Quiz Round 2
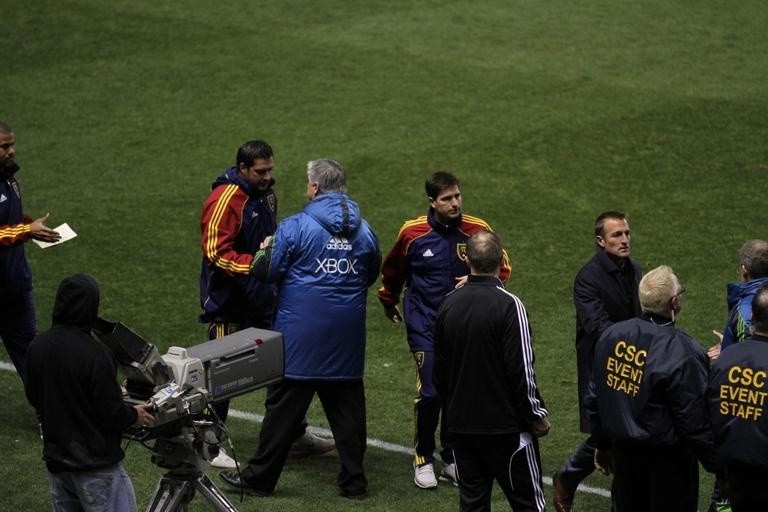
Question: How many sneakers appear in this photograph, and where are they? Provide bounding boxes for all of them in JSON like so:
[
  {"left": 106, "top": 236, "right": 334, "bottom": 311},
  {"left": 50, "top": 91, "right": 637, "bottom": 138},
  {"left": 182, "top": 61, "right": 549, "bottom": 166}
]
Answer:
[
  {"left": 291, "top": 431, "right": 337, "bottom": 456},
  {"left": 439, "top": 462, "right": 460, "bottom": 487},
  {"left": 207, "top": 447, "right": 240, "bottom": 470},
  {"left": 410, "top": 456, "right": 438, "bottom": 490}
]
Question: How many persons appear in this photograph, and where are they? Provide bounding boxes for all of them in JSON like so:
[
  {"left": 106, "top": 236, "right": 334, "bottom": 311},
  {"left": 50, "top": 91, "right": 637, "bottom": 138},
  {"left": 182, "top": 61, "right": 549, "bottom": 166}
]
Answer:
[
  {"left": 707, "top": 239, "right": 768, "bottom": 512},
  {"left": 217, "top": 158, "right": 382, "bottom": 499},
  {"left": 709, "top": 284, "right": 768, "bottom": 512},
  {"left": 198, "top": 139, "right": 336, "bottom": 468},
  {"left": 585, "top": 266, "right": 727, "bottom": 511},
  {"left": 553, "top": 211, "right": 646, "bottom": 511},
  {"left": 0, "top": 122, "right": 62, "bottom": 440},
  {"left": 378, "top": 171, "right": 513, "bottom": 490},
  {"left": 431, "top": 228, "right": 552, "bottom": 512},
  {"left": 23, "top": 272, "right": 155, "bottom": 512}
]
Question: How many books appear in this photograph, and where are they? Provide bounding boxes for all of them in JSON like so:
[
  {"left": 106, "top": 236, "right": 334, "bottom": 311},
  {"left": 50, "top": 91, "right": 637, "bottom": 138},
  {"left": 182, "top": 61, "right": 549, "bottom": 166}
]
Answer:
[{"left": 32, "top": 221, "right": 78, "bottom": 250}]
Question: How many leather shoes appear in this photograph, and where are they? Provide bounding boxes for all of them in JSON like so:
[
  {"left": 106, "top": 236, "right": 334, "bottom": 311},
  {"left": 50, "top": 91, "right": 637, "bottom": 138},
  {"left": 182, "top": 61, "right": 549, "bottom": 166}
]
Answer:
[
  {"left": 551, "top": 473, "right": 574, "bottom": 512},
  {"left": 219, "top": 470, "right": 272, "bottom": 498},
  {"left": 339, "top": 478, "right": 370, "bottom": 501}
]
